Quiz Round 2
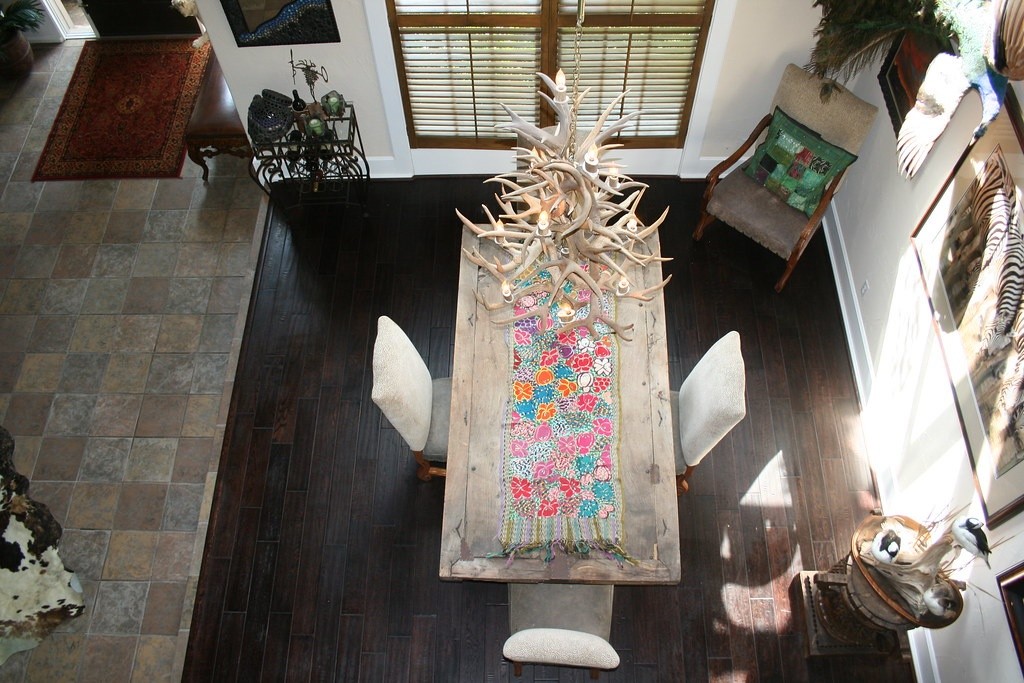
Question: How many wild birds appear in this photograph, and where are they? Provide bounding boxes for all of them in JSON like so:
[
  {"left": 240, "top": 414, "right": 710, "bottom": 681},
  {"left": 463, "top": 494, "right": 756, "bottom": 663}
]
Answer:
[
  {"left": 951, "top": 517, "right": 992, "bottom": 571},
  {"left": 872, "top": 526, "right": 902, "bottom": 564},
  {"left": 923, "top": 582, "right": 961, "bottom": 620}
]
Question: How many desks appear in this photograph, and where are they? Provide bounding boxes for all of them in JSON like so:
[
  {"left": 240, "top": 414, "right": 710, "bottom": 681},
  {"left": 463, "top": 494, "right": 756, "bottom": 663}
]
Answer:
[
  {"left": 439, "top": 222, "right": 682, "bottom": 584},
  {"left": 185, "top": 44, "right": 254, "bottom": 182}
]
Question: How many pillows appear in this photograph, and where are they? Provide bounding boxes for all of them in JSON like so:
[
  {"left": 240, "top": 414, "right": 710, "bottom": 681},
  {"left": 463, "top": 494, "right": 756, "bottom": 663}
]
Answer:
[{"left": 742, "top": 106, "right": 859, "bottom": 221}]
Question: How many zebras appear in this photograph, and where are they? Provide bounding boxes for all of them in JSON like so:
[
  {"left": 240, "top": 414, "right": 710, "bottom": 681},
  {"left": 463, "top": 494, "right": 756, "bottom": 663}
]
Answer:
[{"left": 931, "top": 150, "right": 1024, "bottom": 458}]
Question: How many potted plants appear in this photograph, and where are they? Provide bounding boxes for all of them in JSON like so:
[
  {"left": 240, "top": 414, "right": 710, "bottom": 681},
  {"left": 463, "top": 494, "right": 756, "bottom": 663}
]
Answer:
[{"left": 0, "top": 0, "right": 45, "bottom": 80}]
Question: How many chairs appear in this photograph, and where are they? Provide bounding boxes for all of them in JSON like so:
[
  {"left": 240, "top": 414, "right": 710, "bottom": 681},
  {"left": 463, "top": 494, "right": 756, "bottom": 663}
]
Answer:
[
  {"left": 670, "top": 330, "right": 748, "bottom": 496},
  {"left": 693, "top": 63, "right": 878, "bottom": 293},
  {"left": 502, "top": 583, "right": 621, "bottom": 681},
  {"left": 370, "top": 314, "right": 455, "bottom": 483}
]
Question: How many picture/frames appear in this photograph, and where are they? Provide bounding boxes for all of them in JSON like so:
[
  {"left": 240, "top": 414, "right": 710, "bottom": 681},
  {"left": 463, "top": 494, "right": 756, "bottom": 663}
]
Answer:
[
  {"left": 876, "top": 27, "right": 955, "bottom": 177},
  {"left": 908, "top": 82, "right": 1024, "bottom": 531},
  {"left": 220, "top": 0, "right": 341, "bottom": 48}
]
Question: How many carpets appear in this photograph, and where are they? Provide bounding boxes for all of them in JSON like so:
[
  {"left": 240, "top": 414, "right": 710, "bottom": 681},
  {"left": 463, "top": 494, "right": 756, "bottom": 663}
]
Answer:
[{"left": 31, "top": 35, "right": 216, "bottom": 183}]
